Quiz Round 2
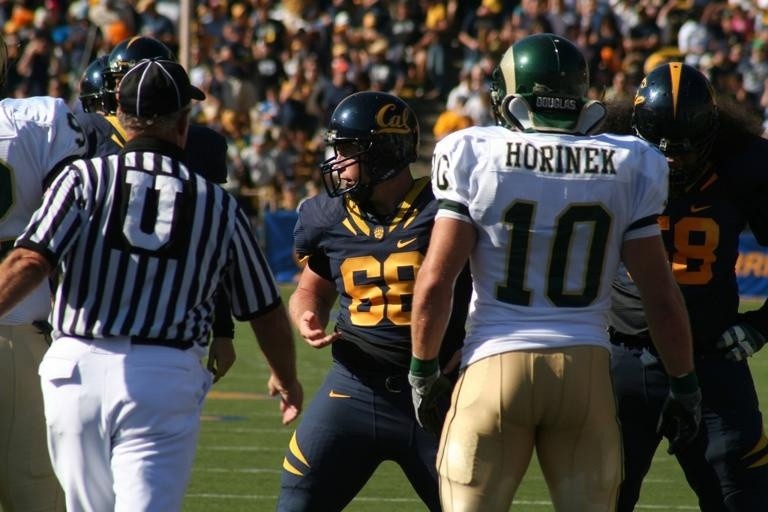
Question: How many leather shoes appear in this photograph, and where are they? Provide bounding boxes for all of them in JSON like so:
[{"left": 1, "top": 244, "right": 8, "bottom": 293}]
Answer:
[
  {"left": 657, "top": 374, "right": 702, "bottom": 454},
  {"left": 720, "top": 299, "right": 767, "bottom": 360},
  {"left": 407, "top": 358, "right": 451, "bottom": 434}
]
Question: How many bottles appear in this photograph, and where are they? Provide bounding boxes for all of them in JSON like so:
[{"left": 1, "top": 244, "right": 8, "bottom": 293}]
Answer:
[{"left": 120, "top": 57, "right": 205, "bottom": 116}]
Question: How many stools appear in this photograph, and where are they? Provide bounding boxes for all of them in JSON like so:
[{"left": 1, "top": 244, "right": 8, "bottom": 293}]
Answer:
[
  {"left": 608, "top": 326, "right": 650, "bottom": 350},
  {"left": 130, "top": 338, "right": 192, "bottom": 350},
  {"left": 378, "top": 373, "right": 406, "bottom": 397}
]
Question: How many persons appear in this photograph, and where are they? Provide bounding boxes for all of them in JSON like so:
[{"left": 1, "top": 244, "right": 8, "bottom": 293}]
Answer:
[
  {"left": 608, "top": 62, "right": 768, "bottom": 512},
  {"left": 80, "top": 56, "right": 118, "bottom": 116},
  {"left": 78, "top": 35, "right": 235, "bottom": 383},
  {"left": 2, "top": 58, "right": 304, "bottom": 512},
  {"left": 277, "top": 92, "right": 472, "bottom": 511},
  {"left": 0, "top": 96, "right": 90, "bottom": 512},
  {"left": 408, "top": 30, "right": 702, "bottom": 512},
  {"left": 0, "top": 0, "right": 767, "bottom": 208}
]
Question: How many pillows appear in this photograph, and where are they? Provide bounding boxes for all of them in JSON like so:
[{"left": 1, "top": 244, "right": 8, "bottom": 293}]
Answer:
[
  {"left": 79, "top": 56, "right": 111, "bottom": 102},
  {"left": 492, "top": 36, "right": 591, "bottom": 115},
  {"left": 107, "top": 37, "right": 177, "bottom": 74},
  {"left": 318, "top": 91, "right": 420, "bottom": 200},
  {"left": 634, "top": 62, "right": 718, "bottom": 146}
]
